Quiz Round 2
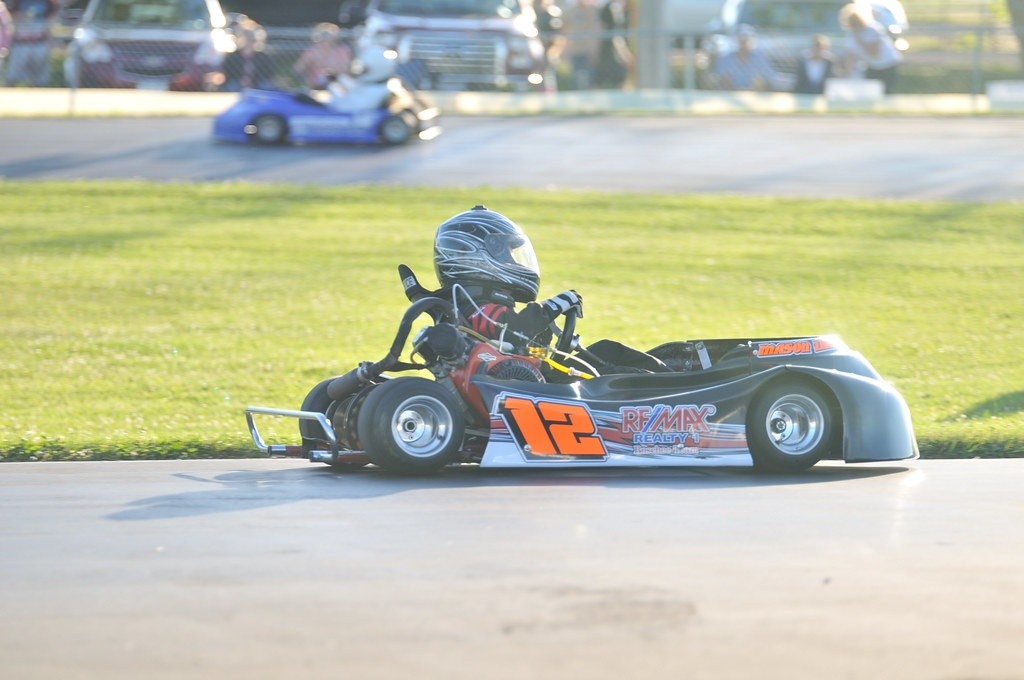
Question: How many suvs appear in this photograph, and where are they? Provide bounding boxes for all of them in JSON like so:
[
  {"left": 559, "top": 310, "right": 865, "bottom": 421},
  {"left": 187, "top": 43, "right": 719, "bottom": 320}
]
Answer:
[{"left": 338, "top": 0, "right": 563, "bottom": 94}]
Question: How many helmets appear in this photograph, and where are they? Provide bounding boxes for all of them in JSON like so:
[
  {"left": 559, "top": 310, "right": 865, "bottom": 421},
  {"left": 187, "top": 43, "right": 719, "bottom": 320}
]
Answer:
[{"left": 433, "top": 205, "right": 541, "bottom": 304}]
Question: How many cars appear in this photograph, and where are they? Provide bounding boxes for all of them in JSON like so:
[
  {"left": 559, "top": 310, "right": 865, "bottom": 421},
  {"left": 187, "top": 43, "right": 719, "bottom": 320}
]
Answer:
[
  {"left": 62, "top": 0, "right": 237, "bottom": 91},
  {"left": 706, "top": 0, "right": 902, "bottom": 96}
]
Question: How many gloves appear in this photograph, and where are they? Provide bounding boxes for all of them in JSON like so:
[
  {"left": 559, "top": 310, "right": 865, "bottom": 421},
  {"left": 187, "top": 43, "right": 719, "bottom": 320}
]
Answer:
[{"left": 541, "top": 290, "right": 583, "bottom": 320}]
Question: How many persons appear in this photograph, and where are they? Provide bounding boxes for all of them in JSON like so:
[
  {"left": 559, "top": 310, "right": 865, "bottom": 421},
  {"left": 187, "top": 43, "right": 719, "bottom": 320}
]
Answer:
[
  {"left": 0, "top": 0, "right": 910, "bottom": 114},
  {"left": 432, "top": 203, "right": 677, "bottom": 385}
]
